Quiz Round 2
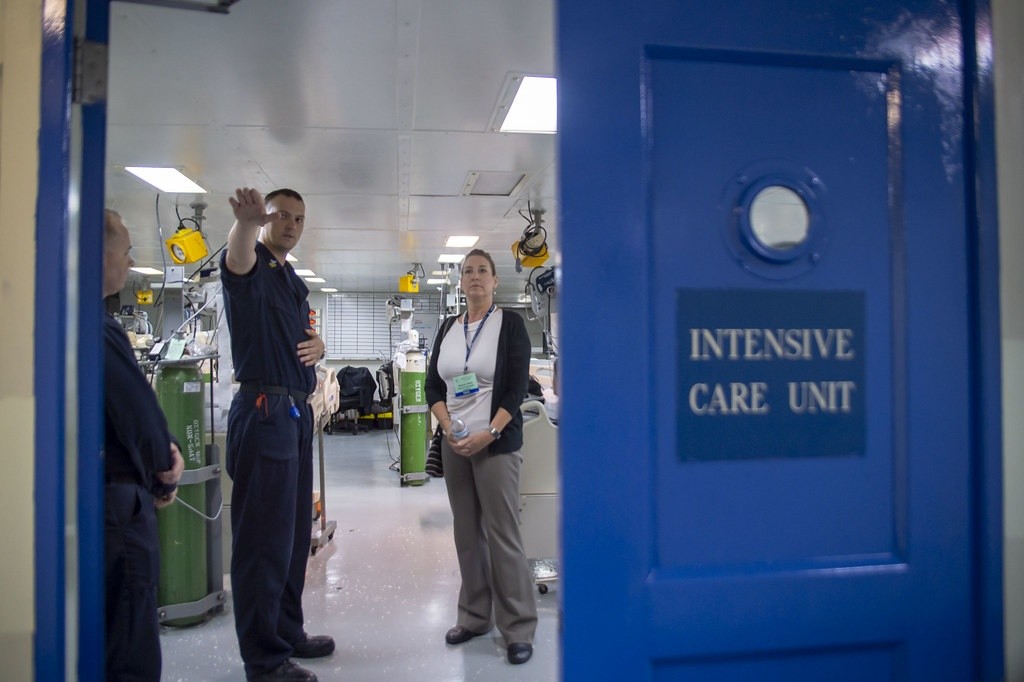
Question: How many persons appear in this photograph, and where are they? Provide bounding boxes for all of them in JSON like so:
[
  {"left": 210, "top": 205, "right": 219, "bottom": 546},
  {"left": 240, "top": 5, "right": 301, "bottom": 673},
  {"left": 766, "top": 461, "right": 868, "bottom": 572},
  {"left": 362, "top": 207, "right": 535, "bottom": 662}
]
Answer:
[
  {"left": 425, "top": 248, "right": 538, "bottom": 664},
  {"left": 219, "top": 186, "right": 335, "bottom": 682},
  {"left": 103, "top": 208, "right": 184, "bottom": 682}
]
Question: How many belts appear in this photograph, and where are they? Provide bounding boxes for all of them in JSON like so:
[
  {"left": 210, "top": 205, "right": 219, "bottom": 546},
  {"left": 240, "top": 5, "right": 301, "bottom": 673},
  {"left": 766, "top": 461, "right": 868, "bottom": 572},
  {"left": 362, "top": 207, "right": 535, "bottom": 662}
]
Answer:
[{"left": 239, "top": 383, "right": 316, "bottom": 404}]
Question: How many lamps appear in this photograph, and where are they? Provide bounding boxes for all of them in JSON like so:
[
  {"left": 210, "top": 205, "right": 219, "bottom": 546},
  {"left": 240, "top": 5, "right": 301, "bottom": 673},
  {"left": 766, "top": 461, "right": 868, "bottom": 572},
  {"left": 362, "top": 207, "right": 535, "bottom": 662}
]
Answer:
[
  {"left": 165, "top": 205, "right": 208, "bottom": 265},
  {"left": 133, "top": 280, "right": 153, "bottom": 304},
  {"left": 399, "top": 263, "right": 425, "bottom": 293},
  {"left": 511, "top": 210, "right": 549, "bottom": 267}
]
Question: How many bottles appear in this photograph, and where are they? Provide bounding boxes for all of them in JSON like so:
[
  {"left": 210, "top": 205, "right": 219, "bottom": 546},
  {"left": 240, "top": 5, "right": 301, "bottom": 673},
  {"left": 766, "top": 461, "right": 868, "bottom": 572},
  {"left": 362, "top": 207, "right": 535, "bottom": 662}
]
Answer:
[{"left": 449, "top": 414, "right": 469, "bottom": 443}]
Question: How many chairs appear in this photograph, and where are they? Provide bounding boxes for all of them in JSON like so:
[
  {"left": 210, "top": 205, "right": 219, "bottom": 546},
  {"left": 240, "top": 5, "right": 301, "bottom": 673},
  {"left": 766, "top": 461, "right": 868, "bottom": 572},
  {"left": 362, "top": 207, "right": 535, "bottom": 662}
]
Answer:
[{"left": 518, "top": 400, "right": 560, "bottom": 595}]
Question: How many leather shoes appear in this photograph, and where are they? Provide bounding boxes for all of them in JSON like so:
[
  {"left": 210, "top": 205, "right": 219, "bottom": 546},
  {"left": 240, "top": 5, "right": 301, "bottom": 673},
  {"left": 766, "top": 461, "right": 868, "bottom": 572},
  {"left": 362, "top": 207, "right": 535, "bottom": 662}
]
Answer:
[
  {"left": 248, "top": 659, "right": 318, "bottom": 682},
  {"left": 446, "top": 625, "right": 485, "bottom": 644},
  {"left": 289, "top": 632, "right": 335, "bottom": 657},
  {"left": 508, "top": 643, "right": 533, "bottom": 663}
]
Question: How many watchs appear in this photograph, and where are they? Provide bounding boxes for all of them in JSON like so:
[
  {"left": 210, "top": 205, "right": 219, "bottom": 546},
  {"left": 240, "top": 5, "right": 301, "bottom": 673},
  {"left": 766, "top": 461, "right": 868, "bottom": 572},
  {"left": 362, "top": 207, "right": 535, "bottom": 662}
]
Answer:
[
  {"left": 487, "top": 426, "right": 501, "bottom": 439},
  {"left": 320, "top": 347, "right": 326, "bottom": 359}
]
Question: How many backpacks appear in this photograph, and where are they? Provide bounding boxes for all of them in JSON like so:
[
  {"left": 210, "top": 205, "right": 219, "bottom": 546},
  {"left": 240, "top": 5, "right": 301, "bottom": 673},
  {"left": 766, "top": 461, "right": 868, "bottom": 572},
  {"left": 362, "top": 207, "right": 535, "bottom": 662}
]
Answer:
[{"left": 377, "top": 362, "right": 396, "bottom": 405}]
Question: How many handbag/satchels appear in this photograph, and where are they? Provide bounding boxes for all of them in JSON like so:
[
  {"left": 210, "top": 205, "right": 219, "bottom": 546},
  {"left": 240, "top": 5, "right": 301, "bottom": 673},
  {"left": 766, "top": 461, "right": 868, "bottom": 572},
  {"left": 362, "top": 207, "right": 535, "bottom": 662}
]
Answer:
[
  {"left": 335, "top": 366, "right": 377, "bottom": 407},
  {"left": 425, "top": 422, "right": 444, "bottom": 477}
]
